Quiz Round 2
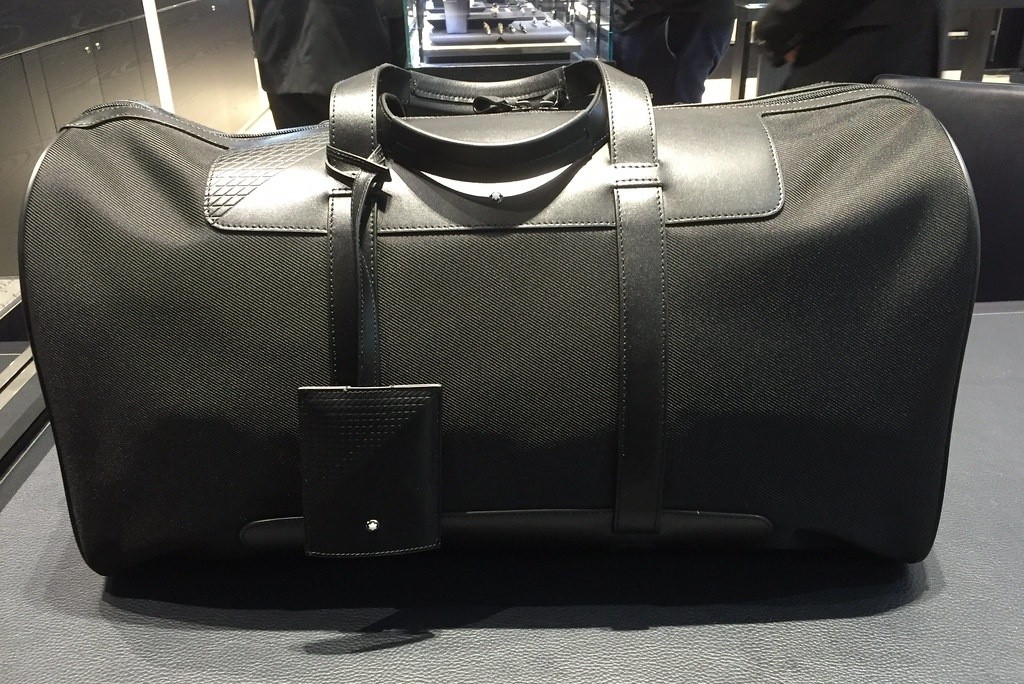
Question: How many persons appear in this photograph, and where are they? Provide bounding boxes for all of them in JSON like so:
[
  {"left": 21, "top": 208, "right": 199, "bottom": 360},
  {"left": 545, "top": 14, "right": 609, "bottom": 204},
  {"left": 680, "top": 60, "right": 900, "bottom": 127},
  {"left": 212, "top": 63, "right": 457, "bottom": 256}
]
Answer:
[
  {"left": 252, "top": 1, "right": 408, "bottom": 130},
  {"left": 610, "top": 0, "right": 736, "bottom": 106},
  {"left": 753, "top": 0, "right": 945, "bottom": 91}
]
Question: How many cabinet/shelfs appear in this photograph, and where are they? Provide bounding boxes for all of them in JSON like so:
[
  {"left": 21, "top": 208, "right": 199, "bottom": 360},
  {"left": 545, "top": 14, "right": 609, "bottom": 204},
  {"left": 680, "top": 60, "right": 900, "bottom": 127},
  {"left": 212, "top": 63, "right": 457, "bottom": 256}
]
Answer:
[{"left": 0, "top": 0, "right": 271, "bottom": 271}]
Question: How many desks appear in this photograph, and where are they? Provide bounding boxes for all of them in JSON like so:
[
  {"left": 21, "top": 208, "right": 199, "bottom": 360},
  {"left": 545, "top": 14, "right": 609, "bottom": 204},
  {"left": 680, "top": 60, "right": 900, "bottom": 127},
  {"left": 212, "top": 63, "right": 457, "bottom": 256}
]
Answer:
[{"left": 705, "top": 0, "right": 770, "bottom": 101}]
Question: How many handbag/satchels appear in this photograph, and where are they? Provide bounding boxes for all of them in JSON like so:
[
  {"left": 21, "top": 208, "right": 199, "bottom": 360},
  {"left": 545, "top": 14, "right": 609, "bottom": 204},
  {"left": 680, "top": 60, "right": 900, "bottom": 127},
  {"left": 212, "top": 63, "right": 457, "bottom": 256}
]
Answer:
[{"left": 18, "top": 58, "right": 982, "bottom": 615}]
